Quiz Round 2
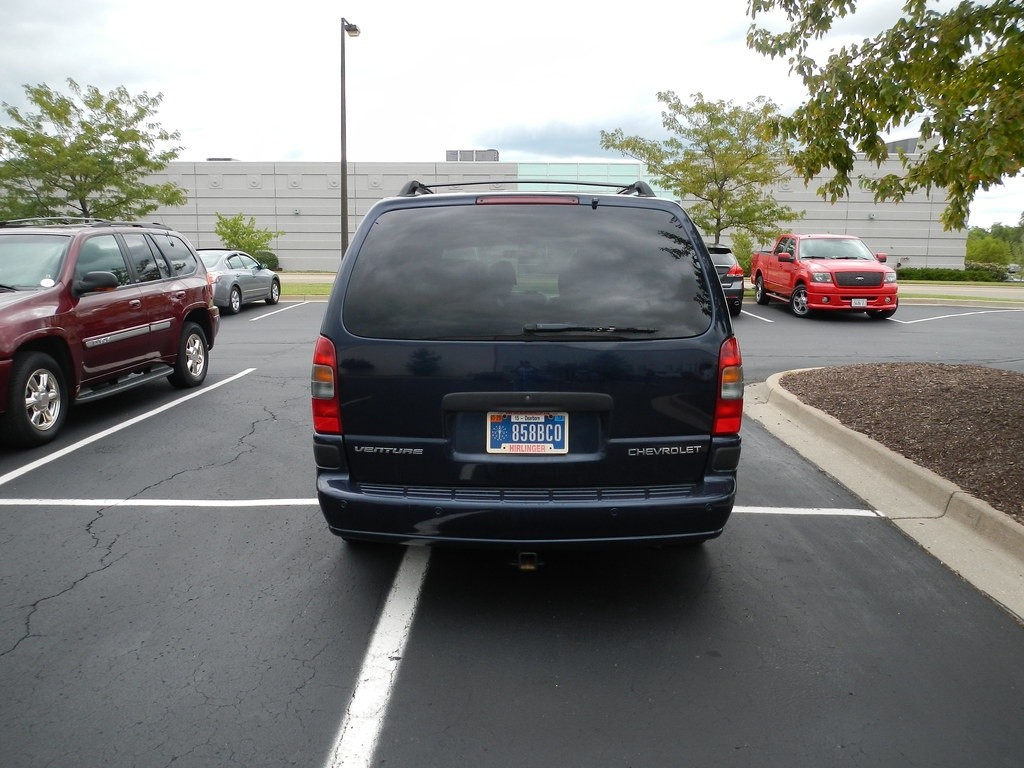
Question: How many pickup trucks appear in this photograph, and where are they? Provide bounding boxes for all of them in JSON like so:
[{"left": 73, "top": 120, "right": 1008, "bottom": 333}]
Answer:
[{"left": 750, "top": 234, "right": 900, "bottom": 320}]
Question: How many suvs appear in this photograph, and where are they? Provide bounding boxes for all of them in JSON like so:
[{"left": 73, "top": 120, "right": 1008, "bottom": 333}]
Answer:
[
  {"left": 307, "top": 177, "right": 744, "bottom": 555},
  {"left": 0, "top": 215, "right": 219, "bottom": 449}
]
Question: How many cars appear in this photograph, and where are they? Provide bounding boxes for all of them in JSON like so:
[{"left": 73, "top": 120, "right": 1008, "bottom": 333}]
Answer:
[
  {"left": 195, "top": 247, "right": 283, "bottom": 313},
  {"left": 704, "top": 245, "right": 745, "bottom": 317}
]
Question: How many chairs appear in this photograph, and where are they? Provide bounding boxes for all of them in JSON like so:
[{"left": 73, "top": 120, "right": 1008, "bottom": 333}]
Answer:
[{"left": 74, "top": 243, "right": 110, "bottom": 280}]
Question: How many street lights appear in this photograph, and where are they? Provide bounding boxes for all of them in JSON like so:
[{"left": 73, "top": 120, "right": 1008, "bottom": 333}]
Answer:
[{"left": 340, "top": 17, "right": 361, "bottom": 264}]
